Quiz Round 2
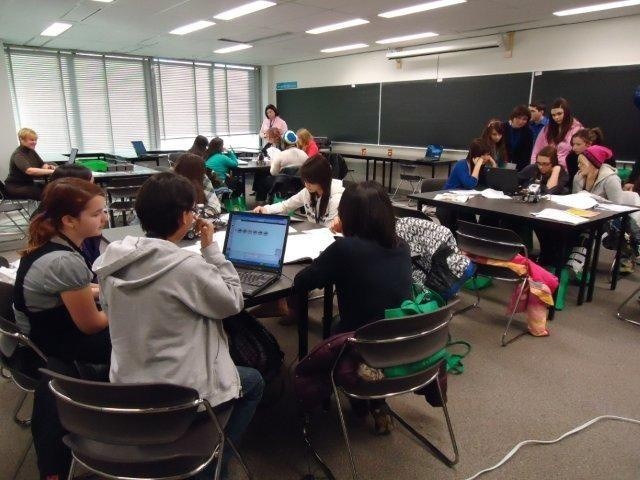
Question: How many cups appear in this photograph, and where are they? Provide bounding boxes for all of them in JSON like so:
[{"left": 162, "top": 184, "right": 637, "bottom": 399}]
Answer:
[
  {"left": 388, "top": 148, "right": 392, "bottom": 156},
  {"left": 361, "top": 148, "right": 366, "bottom": 156}
]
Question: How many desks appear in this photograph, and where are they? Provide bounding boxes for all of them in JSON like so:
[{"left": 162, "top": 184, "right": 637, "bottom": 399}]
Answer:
[
  {"left": 2, "top": 221, "right": 343, "bottom": 372},
  {"left": 407, "top": 188, "right": 640, "bottom": 320}
]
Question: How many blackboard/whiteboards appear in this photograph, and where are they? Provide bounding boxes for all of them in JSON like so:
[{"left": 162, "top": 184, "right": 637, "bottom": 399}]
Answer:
[{"left": 276, "top": 65, "right": 640, "bottom": 164}]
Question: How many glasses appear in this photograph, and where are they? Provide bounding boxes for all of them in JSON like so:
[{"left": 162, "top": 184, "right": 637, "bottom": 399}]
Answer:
[{"left": 535, "top": 162, "right": 552, "bottom": 165}]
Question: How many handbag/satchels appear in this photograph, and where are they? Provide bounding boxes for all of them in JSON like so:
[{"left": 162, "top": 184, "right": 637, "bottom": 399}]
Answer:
[
  {"left": 427, "top": 144, "right": 444, "bottom": 160},
  {"left": 222, "top": 309, "right": 285, "bottom": 405},
  {"left": 384, "top": 293, "right": 446, "bottom": 377}
]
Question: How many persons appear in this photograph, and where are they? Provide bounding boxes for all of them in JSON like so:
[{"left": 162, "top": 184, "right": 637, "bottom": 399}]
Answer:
[
  {"left": 13, "top": 164, "right": 112, "bottom": 364},
  {"left": 436, "top": 97, "right": 640, "bottom": 273},
  {"left": 6, "top": 129, "right": 59, "bottom": 200},
  {"left": 253, "top": 154, "right": 346, "bottom": 326},
  {"left": 175, "top": 135, "right": 239, "bottom": 218},
  {"left": 293, "top": 181, "right": 412, "bottom": 435},
  {"left": 249, "top": 104, "right": 318, "bottom": 206},
  {"left": 92, "top": 172, "right": 264, "bottom": 479}
]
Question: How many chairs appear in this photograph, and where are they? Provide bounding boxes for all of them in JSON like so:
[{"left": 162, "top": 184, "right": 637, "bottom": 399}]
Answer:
[
  {"left": 615, "top": 257, "right": 640, "bottom": 327},
  {"left": 2, "top": 320, "right": 48, "bottom": 430},
  {"left": 300, "top": 301, "right": 464, "bottom": 478},
  {"left": 451, "top": 220, "right": 560, "bottom": 347},
  {"left": 46, "top": 379, "right": 250, "bottom": 478}
]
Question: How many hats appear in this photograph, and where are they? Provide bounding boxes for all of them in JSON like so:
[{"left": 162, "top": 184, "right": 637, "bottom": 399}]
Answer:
[
  {"left": 283, "top": 130, "right": 297, "bottom": 145},
  {"left": 582, "top": 144, "right": 612, "bottom": 169}
]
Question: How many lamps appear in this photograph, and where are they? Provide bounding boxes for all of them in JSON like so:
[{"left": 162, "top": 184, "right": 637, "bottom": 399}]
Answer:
[{"left": 386, "top": 32, "right": 514, "bottom": 69}]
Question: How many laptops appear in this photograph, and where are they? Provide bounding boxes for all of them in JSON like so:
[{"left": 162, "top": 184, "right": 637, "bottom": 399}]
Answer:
[
  {"left": 222, "top": 210, "right": 290, "bottom": 299},
  {"left": 483, "top": 168, "right": 526, "bottom": 192},
  {"left": 131, "top": 140, "right": 160, "bottom": 157},
  {"left": 55, "top": 147, "right": 78, "bottom": 168}
]
